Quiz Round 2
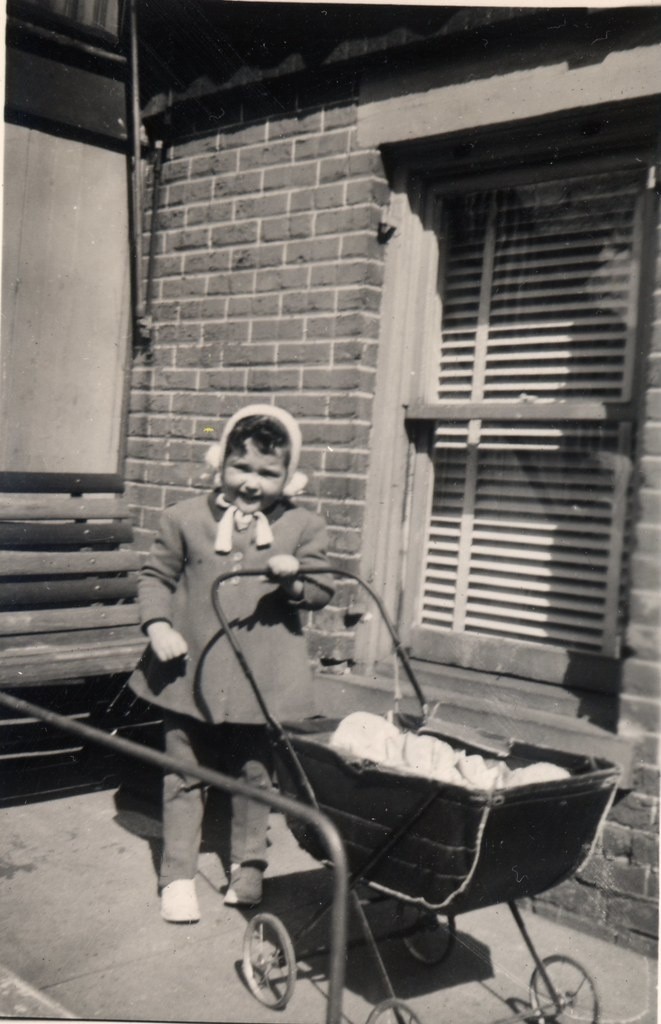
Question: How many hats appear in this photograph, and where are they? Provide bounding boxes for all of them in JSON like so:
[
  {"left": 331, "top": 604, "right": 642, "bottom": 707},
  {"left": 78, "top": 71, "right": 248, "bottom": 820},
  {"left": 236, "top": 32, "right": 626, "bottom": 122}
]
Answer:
[{"left": 200, "top": 401, "right": 310, "bottom": 555}]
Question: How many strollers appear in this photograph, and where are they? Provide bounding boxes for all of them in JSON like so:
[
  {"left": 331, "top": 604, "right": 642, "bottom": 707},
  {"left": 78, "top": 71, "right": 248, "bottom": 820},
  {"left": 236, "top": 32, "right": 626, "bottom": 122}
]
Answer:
[{"left": 209, "top": 567, "right": 623, "bottom": 1023}]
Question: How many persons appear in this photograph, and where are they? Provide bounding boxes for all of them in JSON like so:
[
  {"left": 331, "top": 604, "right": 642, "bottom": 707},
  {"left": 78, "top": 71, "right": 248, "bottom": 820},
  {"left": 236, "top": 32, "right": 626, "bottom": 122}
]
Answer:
[{"left": 128, "top": 404, "right": 335, "bottom": 922}]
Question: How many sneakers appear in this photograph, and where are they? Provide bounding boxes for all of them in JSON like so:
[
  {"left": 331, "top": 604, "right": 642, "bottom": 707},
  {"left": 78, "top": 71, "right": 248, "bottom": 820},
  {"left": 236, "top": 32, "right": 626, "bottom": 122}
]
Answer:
[
  {"left": 160, "top": 878, "right": 201, "bottom": 923},
  {"left": 221, "top": 865, "right": 266, "bottom": 907}
]
